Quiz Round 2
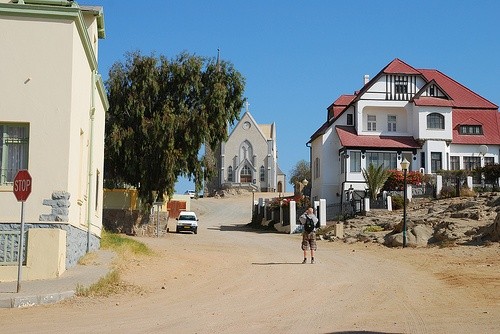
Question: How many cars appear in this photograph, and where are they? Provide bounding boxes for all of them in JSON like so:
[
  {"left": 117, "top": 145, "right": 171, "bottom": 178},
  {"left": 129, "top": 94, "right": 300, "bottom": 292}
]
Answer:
[{"left": 185, "top": 190, "right": 197, "bottom": 199}]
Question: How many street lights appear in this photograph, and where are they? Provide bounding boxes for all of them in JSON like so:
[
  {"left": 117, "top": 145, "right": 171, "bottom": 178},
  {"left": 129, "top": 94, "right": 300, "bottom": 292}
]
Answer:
[
  {"left": 302, "top": 176, "right": 309, "bottom": 212},
  {"left": 201, "top": 167, "right": 205, "bottom": 193},
  {"left": 400, "top": 156, "right": 410, "bottom": 248}
]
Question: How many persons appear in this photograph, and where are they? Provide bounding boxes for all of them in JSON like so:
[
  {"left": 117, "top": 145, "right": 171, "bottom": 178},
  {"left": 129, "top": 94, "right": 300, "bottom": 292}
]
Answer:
[{"left": 299, "top": 207, "right": 318, "bottom": 264}]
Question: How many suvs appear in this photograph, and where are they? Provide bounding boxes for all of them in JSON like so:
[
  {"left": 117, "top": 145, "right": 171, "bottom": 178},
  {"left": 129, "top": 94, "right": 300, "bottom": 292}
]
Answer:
[{"left": 176, "top": 211, "right": 199, "bottom": 233}]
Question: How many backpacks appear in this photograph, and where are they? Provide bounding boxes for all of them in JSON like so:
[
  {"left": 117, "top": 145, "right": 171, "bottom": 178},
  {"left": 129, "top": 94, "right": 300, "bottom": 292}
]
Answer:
[{"left": 304, "top": 215, "right": 314, "bottom": 234}]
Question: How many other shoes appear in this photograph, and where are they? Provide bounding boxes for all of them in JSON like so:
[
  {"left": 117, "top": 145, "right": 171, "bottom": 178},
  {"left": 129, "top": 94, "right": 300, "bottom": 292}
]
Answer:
[
  {"left": 302, "top": 260, "right": 306, "bottom": 264},
  {"left": 311, "top": 259, "right": 314, "bottom": 264}
]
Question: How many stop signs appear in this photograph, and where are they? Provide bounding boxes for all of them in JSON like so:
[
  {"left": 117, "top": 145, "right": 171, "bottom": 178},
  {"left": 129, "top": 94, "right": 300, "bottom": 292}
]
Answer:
[{"left": 13, "top": 169, "right": 33, "bottom": 202}]
